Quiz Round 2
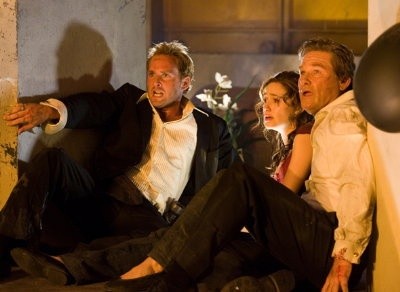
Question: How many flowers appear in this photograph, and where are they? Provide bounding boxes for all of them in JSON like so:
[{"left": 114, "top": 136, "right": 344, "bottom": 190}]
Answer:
[{"left": 197, "top": 71, "right": 241, "bottom": 114}]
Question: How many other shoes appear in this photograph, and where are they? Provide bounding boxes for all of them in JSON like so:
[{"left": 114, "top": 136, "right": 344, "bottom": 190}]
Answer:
[
  {"left": 105, "top": 269, "right": 174, "bottom": 290},
  {"left": 10, "top": 247, "right": 72, "bottom": 287}
]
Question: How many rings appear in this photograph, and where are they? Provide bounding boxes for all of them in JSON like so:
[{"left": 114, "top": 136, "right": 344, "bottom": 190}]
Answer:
[{"left": 20, "top": 106, "right": 24, "bottom": 111}]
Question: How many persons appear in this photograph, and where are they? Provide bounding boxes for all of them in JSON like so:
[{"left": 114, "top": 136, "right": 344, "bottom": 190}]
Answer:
[
  {"left": 0, "top": 39, "right": 241, "bottom": 283},
  {"left": 8, "top": 69, "right": 316, "bottom": 292},
  {"left": 137, "top": 36, "right": 378, "bottom": 292}
]
